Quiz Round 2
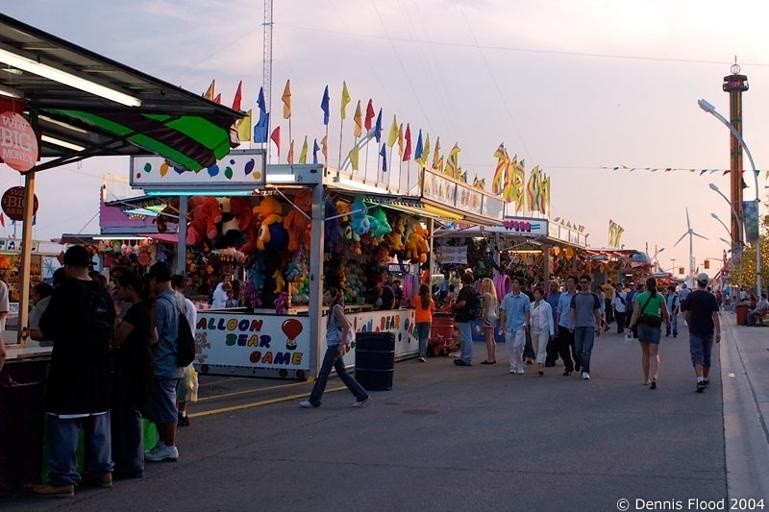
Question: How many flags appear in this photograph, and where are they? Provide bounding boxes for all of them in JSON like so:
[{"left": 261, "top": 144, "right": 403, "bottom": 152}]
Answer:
[
  {"left": 202, "top": 79, "right": 586, "bottom": 233},
  {"left": 607, "top": 220, "right": 625, "bottom": 249}
]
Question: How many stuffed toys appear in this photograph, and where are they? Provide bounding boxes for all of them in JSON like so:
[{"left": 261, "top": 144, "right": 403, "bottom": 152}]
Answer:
[{"left": 156, "top": 190, "right": 432, "bottom": 315}]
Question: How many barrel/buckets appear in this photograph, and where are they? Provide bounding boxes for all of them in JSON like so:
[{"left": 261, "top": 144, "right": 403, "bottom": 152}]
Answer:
[
  {"left": 355, "top": 332, "right": 395, "bottom": 391},
  {"left": 432, "top": 318, "right": 454, "bottom": 346}
]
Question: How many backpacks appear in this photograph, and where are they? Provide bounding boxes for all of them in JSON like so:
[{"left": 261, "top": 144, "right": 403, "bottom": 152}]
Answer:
[{"left": 470, "top": 296, "right": 480, "bottom": 319}]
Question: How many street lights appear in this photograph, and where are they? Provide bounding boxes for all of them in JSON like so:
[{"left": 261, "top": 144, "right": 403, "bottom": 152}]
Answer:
[
  {"left": 698, "top": 97, "right": 764, "bottom": 322},
  {"left": 670, "top": 258, "right": 676, "bottom": 275},
  {"left": 650, "top": 248, "right": 665, "bottom": 261},
  {"left": 339, "top": 125, "right": 384, "bottom": 172},
  {"left": 718, "top": 237, "right": 731, "bottom": 249},
  {"left": 708, "top": 183, "right": 744, "bottom": 296},
  {"left": 711, "top": 211, "right": 740, "bottom": 267}
]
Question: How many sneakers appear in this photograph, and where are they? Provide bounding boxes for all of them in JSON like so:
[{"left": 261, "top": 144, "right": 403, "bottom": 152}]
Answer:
[
  {"left": 300, "top": 400, "right": 320, "bottom": 408},
  {"left": 481, "top": 360, "right": 497, "bottom": 364},
  {"left": 583, "top": 371, "right": 590, "bottom": 380},
  {"left": 144, "top": 442, "right": 179, "bottom": 462},
  {"left": 176, "top": 411, "right": 190, "bottom": 427},
  {"left": 353, "top": 396, "right": 371, "bottom": 408},
  {"left": 33, "top": 484, "right": 75, "bottom": 498},
  {"left": 696, "top": 378, "right": 709, "bottom": 391},
  {"left": 87, "top": 470, "right": 113, "bottom": 486}
]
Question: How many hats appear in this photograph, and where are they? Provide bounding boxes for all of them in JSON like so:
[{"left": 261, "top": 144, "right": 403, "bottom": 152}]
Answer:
[
  {"left": 64, "top": 245, "right": 97, "bottom": 267},
  {"left": 696, "top": 272, "right": 708, "bottom": 282},
  {"left": 339, "top": 323, "right": 355, "bottom": 344}
]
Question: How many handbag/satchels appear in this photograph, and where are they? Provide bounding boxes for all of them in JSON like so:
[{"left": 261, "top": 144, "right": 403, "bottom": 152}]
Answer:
[
  {"left": 630, "top": 324, "right": 637, "bottom": 339},
  {"left": 178, "top": 311, "right": 196, "bottom": 367}
]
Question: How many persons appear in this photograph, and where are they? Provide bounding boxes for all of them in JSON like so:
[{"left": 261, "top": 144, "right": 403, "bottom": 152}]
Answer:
[
  {"left": 411, "top": 284, "right": 436, "bottom": 363},
  {"left": 453, "top": 268, "right": 769, "bottom": 393},
  {"left": 297, "top": 287, "right": 371, "bottom": 409},
  {"left": 0, "top": 244, "right": 199, "bottom": 498}
]
